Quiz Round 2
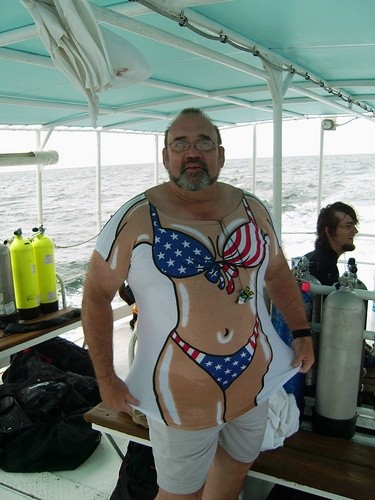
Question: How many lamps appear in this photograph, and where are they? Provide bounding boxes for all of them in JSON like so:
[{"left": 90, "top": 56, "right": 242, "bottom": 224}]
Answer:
[{"left": 321, "top": 116, "right": 360, "bottom": 131}]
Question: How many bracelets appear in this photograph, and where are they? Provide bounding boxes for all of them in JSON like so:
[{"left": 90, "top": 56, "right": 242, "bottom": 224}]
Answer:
[{"left": 292, "top": 328, "right": 313, "bottom": 339}]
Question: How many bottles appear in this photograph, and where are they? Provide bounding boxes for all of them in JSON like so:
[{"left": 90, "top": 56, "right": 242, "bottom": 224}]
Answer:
[{"left": 300, "top": 282, "right": 312, "bottom": 322}]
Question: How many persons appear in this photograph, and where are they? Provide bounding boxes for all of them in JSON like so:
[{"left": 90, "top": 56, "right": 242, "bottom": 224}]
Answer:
[
  {"left": 293, "top": 201, "right": 358, "bottom": 285},
  {"left": 79, "top": 107, "right": 316, "bottom": 500}
]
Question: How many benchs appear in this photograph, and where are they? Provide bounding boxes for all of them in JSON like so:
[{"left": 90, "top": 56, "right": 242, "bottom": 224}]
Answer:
[
  {"left": 0, "top": 273, "right": 83, "bottom": 368},
  {"left": 83, "top": 401, "right": 375, "bottom": 500}
]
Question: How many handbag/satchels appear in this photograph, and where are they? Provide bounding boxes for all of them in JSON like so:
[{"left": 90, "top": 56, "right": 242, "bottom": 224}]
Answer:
[{"left": 0, "top": 336, "right": 102, "bottom": 473}]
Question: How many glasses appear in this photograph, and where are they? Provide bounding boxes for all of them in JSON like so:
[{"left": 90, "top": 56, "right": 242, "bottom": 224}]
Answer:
[{"left": 168, "top": 140, "right": 214, "bottom": 153}]
